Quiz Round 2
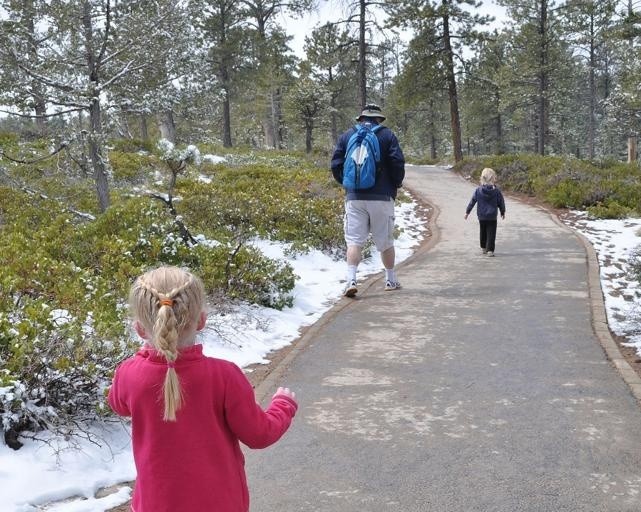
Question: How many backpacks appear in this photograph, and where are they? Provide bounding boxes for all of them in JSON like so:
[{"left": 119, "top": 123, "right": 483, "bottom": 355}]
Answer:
[{"left": 342, "top": 125, "right": 388, "bottom": 191}]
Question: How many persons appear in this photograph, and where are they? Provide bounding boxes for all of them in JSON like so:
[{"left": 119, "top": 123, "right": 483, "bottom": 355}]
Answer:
[
  {"left": 464, "top": 167, "right": 506, "bottom": 257},
  {"left": 331, "top": 102, "right": 406, "bottom": 297},
  {"left": 109, "top": 264, "right": 298, "bottom": 512}
]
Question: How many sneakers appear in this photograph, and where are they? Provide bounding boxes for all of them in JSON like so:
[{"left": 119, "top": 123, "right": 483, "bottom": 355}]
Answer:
[
  {"left": 482, "top": 248, "right": 495, "bottom": 257},
  {"left": 385, "top": 280, "right": 403, "bottom": 291},
  {"left": 342, "top": 280, "right": 358, "bottom": 296}
]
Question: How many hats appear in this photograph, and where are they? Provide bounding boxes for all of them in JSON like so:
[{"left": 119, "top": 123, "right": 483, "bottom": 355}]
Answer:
[{"left": 357, "top": 104, "right": 387, "bottom": 124}]
